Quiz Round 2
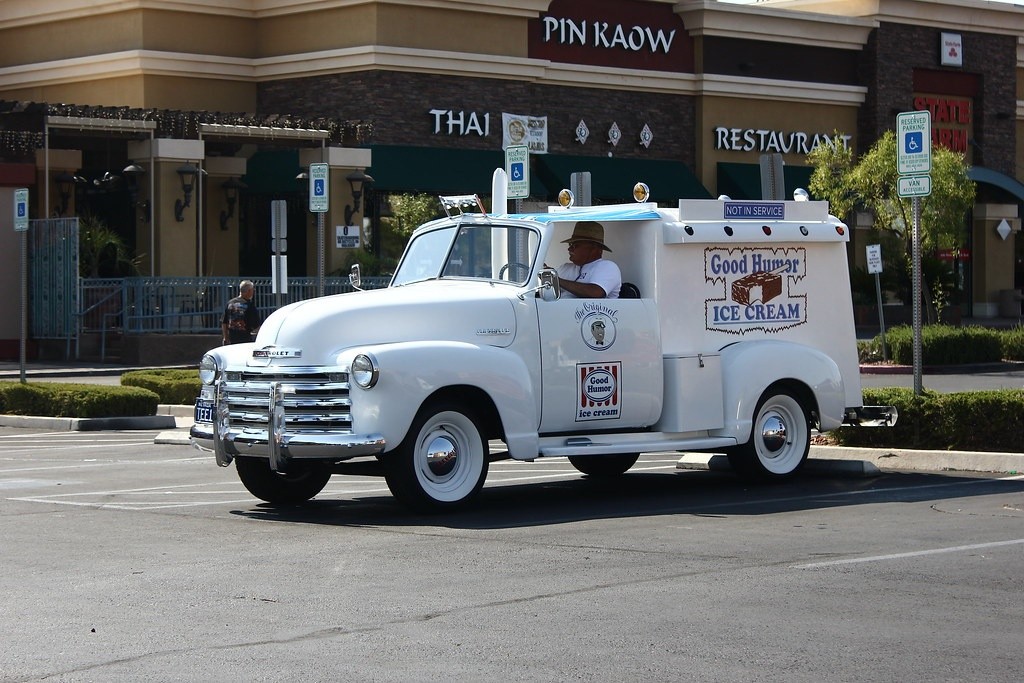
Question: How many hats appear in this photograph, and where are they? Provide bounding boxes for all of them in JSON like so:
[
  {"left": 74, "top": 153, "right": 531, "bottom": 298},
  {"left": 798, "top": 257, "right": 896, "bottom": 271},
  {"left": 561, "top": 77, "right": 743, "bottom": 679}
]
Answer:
[{"left": 560, "top": 221, "right": 613, "bottom": 254}]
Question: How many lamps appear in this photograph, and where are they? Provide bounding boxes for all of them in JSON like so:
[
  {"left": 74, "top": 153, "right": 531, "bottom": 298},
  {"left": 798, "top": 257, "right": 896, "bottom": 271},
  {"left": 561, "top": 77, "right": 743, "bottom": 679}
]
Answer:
[
  {"left": 123, "top": 163, "right": 151, "bottom": 221},
  {"left": 56, "top": 168, "right": 72, "bottom": 210},
  {"left": 220, "top": 177, "right": 248, "bottom": 228},
  {"left": 345, "top": 168, "right": 366, "bottom": 225},
  {"left": 175, "top": 160, "right": 196, "bottom": 222}
]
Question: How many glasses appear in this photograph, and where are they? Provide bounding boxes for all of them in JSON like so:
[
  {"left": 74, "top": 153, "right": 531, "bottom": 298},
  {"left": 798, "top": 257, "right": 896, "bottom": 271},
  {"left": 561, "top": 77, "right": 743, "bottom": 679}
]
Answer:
[{"left": 569, "top": 242, "right": 577, "bottom": 248}]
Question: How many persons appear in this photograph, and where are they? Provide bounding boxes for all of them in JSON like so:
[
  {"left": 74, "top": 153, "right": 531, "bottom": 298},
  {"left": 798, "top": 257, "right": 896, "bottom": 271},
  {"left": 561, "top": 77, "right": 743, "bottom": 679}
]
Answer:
[
  {"left": 543, "top": 221, "right": 623, "bottom": 299},
  {"left": 219, "top": 279, "right": 262, "bottom": 346}
]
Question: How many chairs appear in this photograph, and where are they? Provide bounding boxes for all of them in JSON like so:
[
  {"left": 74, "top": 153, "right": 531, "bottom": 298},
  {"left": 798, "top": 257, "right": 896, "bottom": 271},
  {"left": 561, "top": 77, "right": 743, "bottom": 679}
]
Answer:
[
  {"left": 145, "top": 286, "right": 174, "bottom": 328},
  {"left": 180, "top": 286, "right": 216, "bottom": 333}
]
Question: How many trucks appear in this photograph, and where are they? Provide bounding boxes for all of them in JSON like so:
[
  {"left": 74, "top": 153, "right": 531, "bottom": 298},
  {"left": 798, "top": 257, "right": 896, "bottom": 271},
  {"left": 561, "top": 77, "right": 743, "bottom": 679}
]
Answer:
[{"left": 188, "top": 169, "right": 897, "bottom": 514}]
